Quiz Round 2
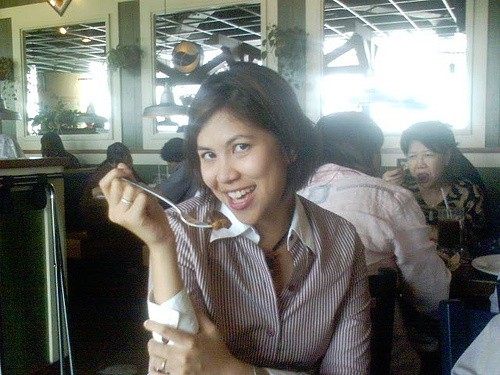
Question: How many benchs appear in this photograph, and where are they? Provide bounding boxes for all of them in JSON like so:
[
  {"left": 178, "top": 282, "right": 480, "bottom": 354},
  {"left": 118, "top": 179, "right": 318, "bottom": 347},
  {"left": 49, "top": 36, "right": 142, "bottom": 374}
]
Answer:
[{"left": 65, "top": 168, "right": 145, "bottom": 278}]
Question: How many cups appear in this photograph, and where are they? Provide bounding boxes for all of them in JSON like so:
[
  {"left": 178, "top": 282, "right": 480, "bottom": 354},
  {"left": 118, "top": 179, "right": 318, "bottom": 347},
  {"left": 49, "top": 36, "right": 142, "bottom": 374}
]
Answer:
[{"left": 438, "top": 208, "right": 462, "bottom": 254}]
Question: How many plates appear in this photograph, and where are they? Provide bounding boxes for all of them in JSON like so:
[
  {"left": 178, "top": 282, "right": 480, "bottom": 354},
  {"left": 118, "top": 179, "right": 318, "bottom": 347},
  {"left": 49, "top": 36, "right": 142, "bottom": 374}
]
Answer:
[{"left": 472, "top": 254, "right": 500, "bottom": 276}]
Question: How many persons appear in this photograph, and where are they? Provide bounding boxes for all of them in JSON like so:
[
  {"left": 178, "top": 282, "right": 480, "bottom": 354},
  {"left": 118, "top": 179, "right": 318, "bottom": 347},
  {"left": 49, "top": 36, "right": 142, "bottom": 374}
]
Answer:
[
  {"left": 146, "top": 137, "right": 202, "bottom": 210},
  {"left": 40, "top": 132, "right": 83, "bottom": 231},
  {"left": 278, "top": 111, "right": 451, "bottom": 316},
  {"left": 380, "top": 119, "right": 487, "bottom": 243},
  {"left": 99, "top": 61, "right": 373, "bottom": 375},
  {"left": 75, "top": 142, "right": 147, "bottom": 263}
]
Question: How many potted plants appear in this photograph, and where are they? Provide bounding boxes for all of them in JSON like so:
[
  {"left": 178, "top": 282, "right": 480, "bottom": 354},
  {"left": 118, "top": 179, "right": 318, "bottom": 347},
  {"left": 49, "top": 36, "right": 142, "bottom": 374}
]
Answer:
[
  {"left": 0, "top": 56, "right": 15, "bottom": 82},
  {"left": 31, "top": 95, "right": 81, "bottom": 134},
  {"left": 261, "top": 24, "right": 310, "bottom": 89},
  {"left": 108, "top": 45, "right": 145, "bottom": 81}
]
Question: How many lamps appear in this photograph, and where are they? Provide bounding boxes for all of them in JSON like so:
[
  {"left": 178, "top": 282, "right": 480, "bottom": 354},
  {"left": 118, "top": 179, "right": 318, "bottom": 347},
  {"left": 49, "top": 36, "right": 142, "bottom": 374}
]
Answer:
[
  {"left": 78, "top": 104, "right": 107, "bottom": 123},
  {"left": 142, "top": 0, "right": 190, "bottom": 115},
  {"left": 155, "top": 117, "right": 178, "bottom": 126}
]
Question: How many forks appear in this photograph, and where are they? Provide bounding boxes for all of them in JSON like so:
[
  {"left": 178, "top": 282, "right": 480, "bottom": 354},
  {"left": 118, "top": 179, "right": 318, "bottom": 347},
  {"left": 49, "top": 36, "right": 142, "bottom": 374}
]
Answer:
[{"left": 120, "top": 177, "right": 212, "bottom": 228}]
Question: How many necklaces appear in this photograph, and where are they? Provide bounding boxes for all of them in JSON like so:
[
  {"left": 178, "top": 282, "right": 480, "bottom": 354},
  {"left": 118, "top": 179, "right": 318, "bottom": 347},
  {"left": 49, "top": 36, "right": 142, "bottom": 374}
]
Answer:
[{"left": 245, "top": 205, "right": 296, "bottom": 278}]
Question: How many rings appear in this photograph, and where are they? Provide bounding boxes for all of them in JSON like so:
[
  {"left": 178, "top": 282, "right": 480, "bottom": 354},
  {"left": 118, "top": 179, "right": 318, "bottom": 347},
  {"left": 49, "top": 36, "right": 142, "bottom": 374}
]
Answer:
[{"left": 153, "top": 359, "right": 168, "bottom": 372}]
